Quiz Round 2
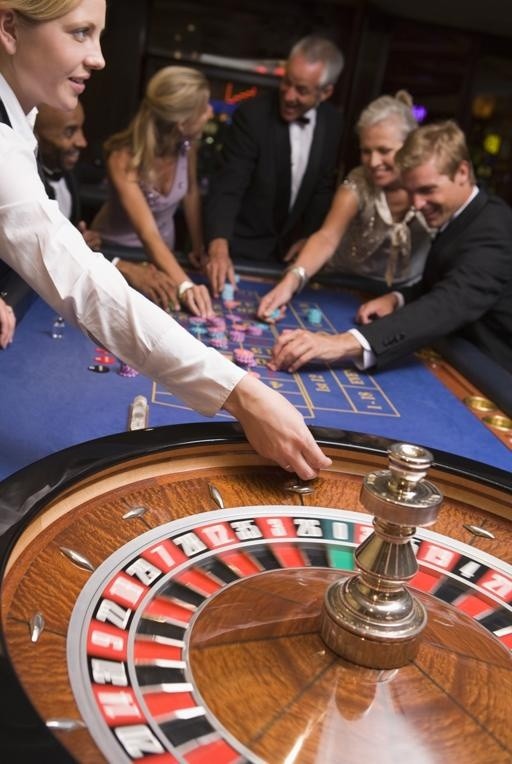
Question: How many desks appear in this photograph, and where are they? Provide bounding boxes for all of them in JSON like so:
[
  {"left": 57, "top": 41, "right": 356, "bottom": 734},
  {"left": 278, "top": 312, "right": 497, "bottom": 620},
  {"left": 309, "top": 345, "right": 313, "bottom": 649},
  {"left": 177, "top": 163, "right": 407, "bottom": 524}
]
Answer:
[{"left": 2, "top": 236, "right": 512, "bottom": 479}]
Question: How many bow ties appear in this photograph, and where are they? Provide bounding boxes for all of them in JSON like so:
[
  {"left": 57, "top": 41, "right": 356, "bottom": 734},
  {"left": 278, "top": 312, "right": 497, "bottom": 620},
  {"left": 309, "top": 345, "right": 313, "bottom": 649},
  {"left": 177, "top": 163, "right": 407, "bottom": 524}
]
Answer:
[{"left": 292, "top": 117, "right": 310, "bottom": 128}]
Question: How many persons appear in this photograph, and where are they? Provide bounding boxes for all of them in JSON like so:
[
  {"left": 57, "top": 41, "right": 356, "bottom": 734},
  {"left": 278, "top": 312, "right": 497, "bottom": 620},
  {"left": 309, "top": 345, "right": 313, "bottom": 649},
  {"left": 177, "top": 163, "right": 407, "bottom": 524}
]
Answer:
[
  {"left": 0, "top": 1, "right": 335, "bottom": 485},
  {"left": 90, "top": 64, "right": 215, "bottom": 320},
  {"left": 255, "top": 90, "right": 443, "bottom": 328},
  {"left": 265, "top": 118, "right": 512, "bottom": 375},
  {"left": 204, "top": 35, "right": 347, "bottom": 303},
  {"left": 33, "top": 98, "right": 103, "bottom": 255}
]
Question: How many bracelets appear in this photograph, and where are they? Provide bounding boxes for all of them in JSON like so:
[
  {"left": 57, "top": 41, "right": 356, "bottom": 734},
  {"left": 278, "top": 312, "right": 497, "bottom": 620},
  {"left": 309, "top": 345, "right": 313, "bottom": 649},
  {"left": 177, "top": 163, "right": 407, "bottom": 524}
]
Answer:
[
  {"left": 281, "top": 263, "right": 309, "bottom": 295},
  {"left": 177, "top": 281, "right": 196, "bottom": 300}
]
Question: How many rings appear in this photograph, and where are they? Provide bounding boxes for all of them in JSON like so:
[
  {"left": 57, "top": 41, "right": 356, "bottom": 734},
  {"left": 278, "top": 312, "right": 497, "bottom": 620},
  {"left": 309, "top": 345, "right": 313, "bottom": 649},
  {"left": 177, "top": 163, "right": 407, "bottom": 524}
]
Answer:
[
  {"left": 6, "top": 303, "right": 15, "bottom": 313},
  {"left": 281, "top": 464, "right": 292, "bottom": 472}
]
